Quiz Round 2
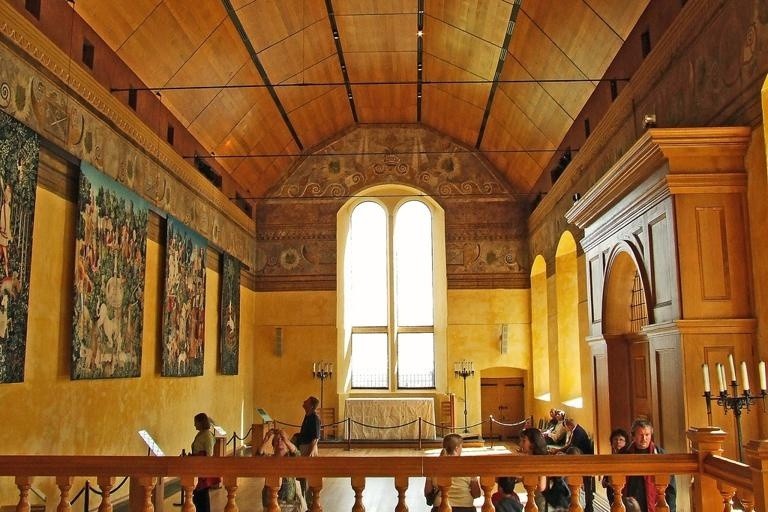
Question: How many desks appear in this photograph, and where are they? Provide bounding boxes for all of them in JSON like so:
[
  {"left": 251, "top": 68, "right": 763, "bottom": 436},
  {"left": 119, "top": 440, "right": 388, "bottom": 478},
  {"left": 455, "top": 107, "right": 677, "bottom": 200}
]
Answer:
[{"left": 344, "top": 397, "right": 436, "bottom": 440}]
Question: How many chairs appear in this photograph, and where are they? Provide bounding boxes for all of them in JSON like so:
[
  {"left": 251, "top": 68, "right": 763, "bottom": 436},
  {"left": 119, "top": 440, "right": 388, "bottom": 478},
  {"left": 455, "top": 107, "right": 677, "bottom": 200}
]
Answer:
[
  {"left": 316, "top": 407, "right": 337, "bottom": 439},
  {"left": 440, "top": 401, "right": 452, "bottom": 438}
]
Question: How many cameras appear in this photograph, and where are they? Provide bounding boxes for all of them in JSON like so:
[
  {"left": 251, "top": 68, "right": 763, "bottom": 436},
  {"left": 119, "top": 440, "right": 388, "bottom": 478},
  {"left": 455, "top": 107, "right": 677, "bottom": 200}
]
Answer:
[{"left": 273, "top": 429, "right": 280, "bottom": 433}]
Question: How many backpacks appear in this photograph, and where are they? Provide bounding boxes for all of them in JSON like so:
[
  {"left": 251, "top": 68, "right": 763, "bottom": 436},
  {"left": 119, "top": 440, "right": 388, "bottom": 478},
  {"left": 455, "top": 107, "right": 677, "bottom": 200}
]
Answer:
[{"left": 540, "top": 473, "right": 573, "bottom": 509}]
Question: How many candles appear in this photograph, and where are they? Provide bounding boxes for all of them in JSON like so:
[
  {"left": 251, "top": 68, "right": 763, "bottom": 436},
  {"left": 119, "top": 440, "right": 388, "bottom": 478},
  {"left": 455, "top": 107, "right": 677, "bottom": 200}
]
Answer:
[
  {"left": 454, "top": 361, "right": 473, "bottom": 371},
  {"left": 702, "top": 353, "right": 767, "bottom": 394},
  {"left": 313, "top": 361, "right": 333, "bottom": 374}
]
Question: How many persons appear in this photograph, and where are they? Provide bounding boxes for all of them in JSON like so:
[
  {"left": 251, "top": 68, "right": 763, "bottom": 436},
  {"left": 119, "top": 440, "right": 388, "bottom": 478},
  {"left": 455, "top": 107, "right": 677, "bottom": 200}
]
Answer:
[
  {"left": 615, "top": 417, "right": 677, "bottom": 512},
  {"left": 297, "top": 395, "right": 321, "bottom": 456},
  {"left": 545, "top": 410, "right": 567, "bottom": 445},
  {"left": 538, "top": 407, "right": 558, "bottom": 438},
  {"left": 600, "top": 428, "right": 632, "bottom": 507},
  {"left": 553, "top": 417, "right": 594, "bottom": 455},
  {"left": 424, "top": 433, "right": 482, "bottom": 512},
  {"left": 513, "top": 427, "right": 550, "bottom": 512},
  {"left": 186, "top": 412, "right": 216, "bottom": 512},
  {"left": 490, "top": 476, "right": 525, "bottom": 512}
]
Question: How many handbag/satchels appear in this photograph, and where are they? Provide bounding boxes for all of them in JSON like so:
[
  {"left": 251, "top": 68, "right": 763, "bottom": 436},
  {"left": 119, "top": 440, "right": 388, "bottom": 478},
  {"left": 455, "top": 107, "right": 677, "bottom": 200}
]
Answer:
[{"left": 425, "top": 487, "right": 440, "bottom": 505}]
